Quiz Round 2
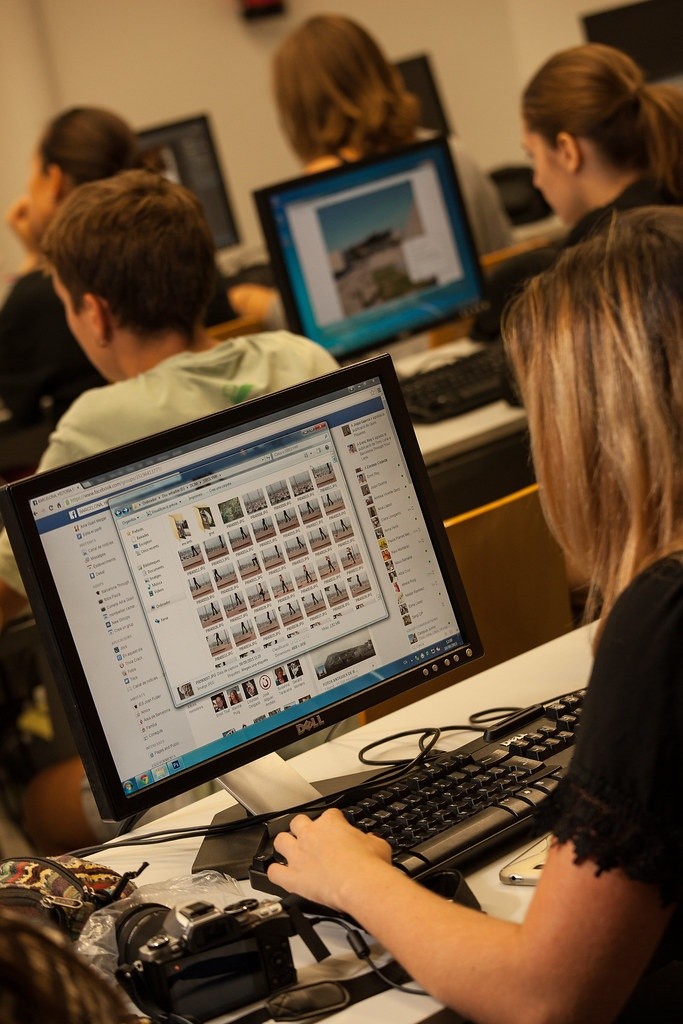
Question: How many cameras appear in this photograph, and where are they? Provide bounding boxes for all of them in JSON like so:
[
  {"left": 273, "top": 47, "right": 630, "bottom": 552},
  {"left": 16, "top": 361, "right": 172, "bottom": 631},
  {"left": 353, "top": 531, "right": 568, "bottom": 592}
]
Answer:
[{"left": 115, "top": 897, "right": 298, "bottom": 1024}]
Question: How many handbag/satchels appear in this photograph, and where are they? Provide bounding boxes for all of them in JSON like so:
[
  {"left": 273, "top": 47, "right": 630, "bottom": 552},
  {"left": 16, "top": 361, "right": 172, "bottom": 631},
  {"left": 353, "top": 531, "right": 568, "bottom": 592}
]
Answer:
[{"left": 0, "top": 854, "right": 149, "bottom": 941}]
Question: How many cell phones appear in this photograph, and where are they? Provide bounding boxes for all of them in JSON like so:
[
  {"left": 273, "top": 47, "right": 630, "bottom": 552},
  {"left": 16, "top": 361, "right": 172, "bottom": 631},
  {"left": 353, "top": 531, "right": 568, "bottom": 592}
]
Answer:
[{"left": 499, "top": 831, "right": 553, "bottom": 887}]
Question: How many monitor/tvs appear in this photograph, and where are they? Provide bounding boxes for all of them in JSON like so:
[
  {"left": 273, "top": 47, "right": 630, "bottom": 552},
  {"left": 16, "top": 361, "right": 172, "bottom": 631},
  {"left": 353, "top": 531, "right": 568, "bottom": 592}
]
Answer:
[
  {"left": 134, "top": 112, "right": 243, "bottom": 254},
  {"left": 579, "top": 0, "right": 683, "bottom": 86},
  {"left": 0, "top": 352, "right": 487, "bottom": 824},
  {"left": 253, "top": 134, "right": 489, "bottom": 367},
  {"left": 392, "top": 50, "right": 455, "bottom": 137}
]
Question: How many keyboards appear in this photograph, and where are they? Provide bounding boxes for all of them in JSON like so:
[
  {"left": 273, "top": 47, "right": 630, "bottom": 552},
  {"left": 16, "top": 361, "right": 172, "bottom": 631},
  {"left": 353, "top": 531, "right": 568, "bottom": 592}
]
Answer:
[
  {"left": 400, "top": 340, "right": 508, "bottom": 426},
  {"left": 251, "top": 685, "right": 588, "bottom": 928}
]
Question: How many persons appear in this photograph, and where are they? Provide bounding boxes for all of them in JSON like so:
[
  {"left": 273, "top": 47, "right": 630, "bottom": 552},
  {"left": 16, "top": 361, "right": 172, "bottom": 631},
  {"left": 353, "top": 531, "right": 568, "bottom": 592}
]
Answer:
[
  {"left": 17, "top": 171, "right": 340, "bottom": 853},
  {"left": 188, "top": 491, "right": 368, "bottom": 710},
  {"left": 472, "top": 46, "right": 683, "bottom": 339},
  {"left": 266, "top": 203, "right": 680, "bottom": 1024},
  {"left": 271, "top": 12, "right": 444, "bottom": 173},
  {"left": 0, "top": 105, "right": 235, "bottom": 413}
]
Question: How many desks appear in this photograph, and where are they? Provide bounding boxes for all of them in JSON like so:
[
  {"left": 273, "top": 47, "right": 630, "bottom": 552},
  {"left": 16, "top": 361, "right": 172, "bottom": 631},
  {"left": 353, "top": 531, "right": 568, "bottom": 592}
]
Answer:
[
  {"left": 1, "top": 335, "right": 525, "bottom": 654},
  {"left": 73, "top": 614, "right": 604, "bottom": 1023}
]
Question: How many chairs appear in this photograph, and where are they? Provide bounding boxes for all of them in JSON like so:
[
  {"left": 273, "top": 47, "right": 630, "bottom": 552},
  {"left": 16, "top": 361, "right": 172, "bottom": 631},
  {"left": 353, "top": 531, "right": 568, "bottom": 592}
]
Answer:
[{"left": 361, "top": 480, "right": 571, "bottom": 728}]
